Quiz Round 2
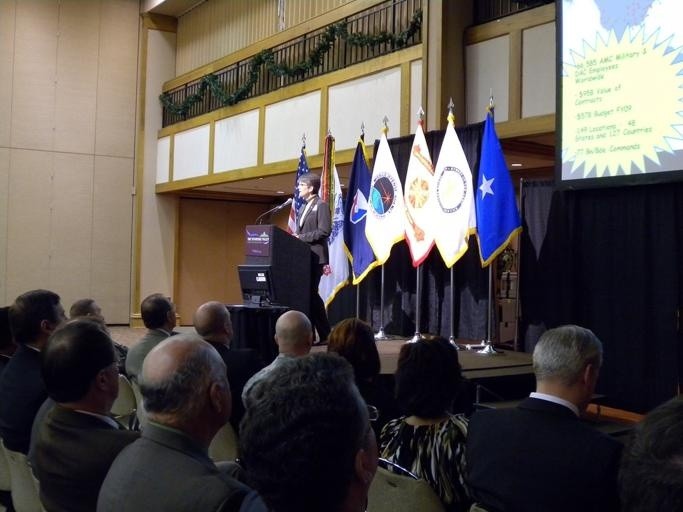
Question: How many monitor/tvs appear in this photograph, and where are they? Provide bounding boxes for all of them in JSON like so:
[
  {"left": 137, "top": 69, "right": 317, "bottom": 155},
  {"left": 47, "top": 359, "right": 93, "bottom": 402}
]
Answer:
[{"left": 236, "top": 263, "right": 273, "bottom": 307}]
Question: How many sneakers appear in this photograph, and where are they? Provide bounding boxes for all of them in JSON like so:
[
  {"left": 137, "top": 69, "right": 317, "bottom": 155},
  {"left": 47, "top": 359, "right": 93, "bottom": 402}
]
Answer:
[{"left": 317, "top": 340, "right": 332, "bottom": 346}]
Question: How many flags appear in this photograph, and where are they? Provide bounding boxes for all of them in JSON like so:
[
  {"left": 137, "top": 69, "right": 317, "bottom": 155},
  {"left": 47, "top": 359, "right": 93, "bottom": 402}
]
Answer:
[
  {"left": 287, "top": 142, "right": 310, "bottom": 236},
  {"left": 402, "top": 121, "right": 435, "bottom": 268},
  {"left": 472, "top": 105, "right": 523, "bottom": 269},
  {"left": 365, "top": 127, "right": 406, "bottom": 266},
  {"left": 429, "top": 113, "right": 477, "bottom": 269},
  {"left": 343, "top": 132, "right": 380, "bottom": 286},
  {"left": 315, "top": 136, "right": 350, "bottom": 308}
]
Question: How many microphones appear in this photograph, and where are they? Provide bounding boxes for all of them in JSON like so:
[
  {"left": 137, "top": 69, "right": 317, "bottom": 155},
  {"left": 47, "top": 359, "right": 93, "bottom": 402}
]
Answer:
[{"left": 272, "top": 198, "right": 293, "bottom": 217}]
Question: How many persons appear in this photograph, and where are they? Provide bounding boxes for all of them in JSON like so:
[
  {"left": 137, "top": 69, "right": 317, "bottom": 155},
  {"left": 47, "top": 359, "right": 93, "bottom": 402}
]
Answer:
[
  {"left": 463, "top": 325, "right": 624, "bottom": 512},
  {"left": 191, "top": 299, "right": 264, "bottom": 421},
  {"left": 241, "top": 309, "right": 313, "bottom": 408},
  {"left": 29, "top": 317, "right": 142, "bottom": 510},
  {"left": 96, "top": 336, "right": 266, "bottom": 510},
  {"left": 3, "top": 290, "right": 69, "bottom": 453},
  {"left": 377, "top": 336, "right": 470, "bottom": 511},
  {"left": 68, "top": 298, "right": 128, "bottom": 375},
  {"left": 236, "top": 353, "right": 378, "bottom": 512},
  {"left": 125, "top": 292, "right": 176, "bottom": 380},
  {"left": 291, "top": 172, "right": 332, "bottom": 346},
  {"left": 325, "top": 318, "right": 382, "bottom": 404}
]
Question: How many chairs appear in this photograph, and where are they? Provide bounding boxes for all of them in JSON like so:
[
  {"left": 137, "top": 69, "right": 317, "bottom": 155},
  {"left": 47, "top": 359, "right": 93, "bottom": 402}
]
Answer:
[
  {"left": 2, "top": 439, "right": 52, "bottom": 512},
  {"left": 370, "top": 454, "right": 447, "bottom": 511},
  {"left": 199, "top": 421, "right": 240, "bottom": 465},
  {"left": 110, "top": 375, "right": 137, "bottom": 430},
  {"left": 1, "top": 437, "right": 14, "bottom": 492}
]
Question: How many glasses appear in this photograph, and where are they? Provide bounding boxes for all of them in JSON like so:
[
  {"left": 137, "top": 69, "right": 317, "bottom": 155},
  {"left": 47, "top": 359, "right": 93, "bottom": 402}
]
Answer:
[{"left": 364, "top": 404, "right": 380, "bottom": 422}]
